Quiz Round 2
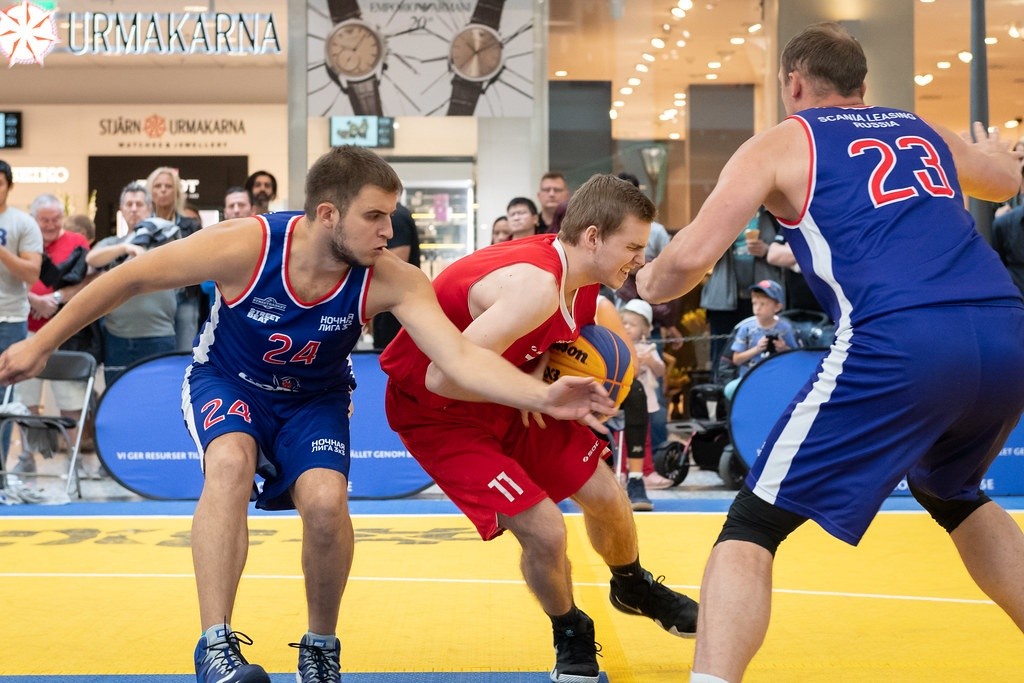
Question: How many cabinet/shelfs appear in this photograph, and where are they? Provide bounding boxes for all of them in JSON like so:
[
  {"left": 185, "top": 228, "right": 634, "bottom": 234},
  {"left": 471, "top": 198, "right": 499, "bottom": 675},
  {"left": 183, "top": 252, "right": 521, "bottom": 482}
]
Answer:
[{"left": 357, "top": 179, "right": 477, "bottom": 349}]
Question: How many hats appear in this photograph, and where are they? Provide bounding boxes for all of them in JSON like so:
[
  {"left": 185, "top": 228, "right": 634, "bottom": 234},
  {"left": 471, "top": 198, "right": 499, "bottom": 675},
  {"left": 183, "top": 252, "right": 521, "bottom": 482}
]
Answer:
[
  {"left": 749, "top": 280, "right": 784, "bottom": 304},
  {"left": 622, "top": 299, "right": 653, "bottom": 333}
]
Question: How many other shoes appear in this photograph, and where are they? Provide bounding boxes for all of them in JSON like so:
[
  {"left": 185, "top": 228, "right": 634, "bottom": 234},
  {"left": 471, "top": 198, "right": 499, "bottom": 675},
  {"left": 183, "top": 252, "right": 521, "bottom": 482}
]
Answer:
[{"left": 13, "top": 458, "right": 35, "bottom": 478}]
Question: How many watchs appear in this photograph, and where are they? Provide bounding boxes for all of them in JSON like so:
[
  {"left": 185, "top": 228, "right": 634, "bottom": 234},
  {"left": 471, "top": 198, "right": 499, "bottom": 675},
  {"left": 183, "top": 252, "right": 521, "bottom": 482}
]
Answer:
[
  {"left": 326, "top": 0, "right": 389, "bottom": 115},
  {"left": 447, "top": 0, "right": 504, "bottom": 116}
]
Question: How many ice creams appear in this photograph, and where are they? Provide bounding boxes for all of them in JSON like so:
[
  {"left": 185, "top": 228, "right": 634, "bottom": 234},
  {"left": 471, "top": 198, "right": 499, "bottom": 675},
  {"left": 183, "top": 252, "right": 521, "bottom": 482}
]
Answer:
[{"left": 745, "top": 228, "right": 761, "bottom": 242}]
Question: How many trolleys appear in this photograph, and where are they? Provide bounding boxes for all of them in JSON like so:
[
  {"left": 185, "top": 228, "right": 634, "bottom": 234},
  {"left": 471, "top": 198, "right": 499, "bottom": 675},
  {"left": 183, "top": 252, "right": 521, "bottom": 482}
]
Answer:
[{"left": 652, "top": 308, "right": 829, "bottom": 491}]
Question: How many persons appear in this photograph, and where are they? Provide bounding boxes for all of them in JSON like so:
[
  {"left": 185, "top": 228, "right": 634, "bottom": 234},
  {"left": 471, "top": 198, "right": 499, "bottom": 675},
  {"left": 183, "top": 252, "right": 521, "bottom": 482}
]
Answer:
[
  {"left": 537, "top": 172, "right": 567, "bottom": 234},
  {"left": 11, "top": 194, "right": 92, "bottom": 479},
  {"left": 636, "top": 23, "right": 1024, "bottom": 683},
  {"left": 546, "top": 201, "right": 569, "bottom": 235},
  {"left": 989, "top": 167, "right": 1024, "bottom": 285},
  {"left": 0, "top": 160, "right": 49, "bottom": 506},
  {"left": 0, "top": 143, "right": 619, "bottom": 683},
  {"left": 63, "top": 167, "right": 278, "bottom": 480},
  {"left": 379, "top": 173, "right": 698, "bottom": 683},
  {"left": 505, "top": 198, "right": 540, "bottom": 241},
  {"left": 491, "top": 216, "right": 513, "bottom": 245},
  {"left": 619, "top": 172, "right": 834, "bottom": 510},
  {"left": 372, "top": 202, "right": 420, "bottom": 350}
]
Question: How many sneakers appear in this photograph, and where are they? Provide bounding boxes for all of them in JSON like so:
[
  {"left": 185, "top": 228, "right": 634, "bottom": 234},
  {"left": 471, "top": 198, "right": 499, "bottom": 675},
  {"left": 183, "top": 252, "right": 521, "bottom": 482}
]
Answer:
[
  {"left": 194, "top": 615, "right": 272, "bottom": 683},
  {"left": 288, "top": 633, "right": 341, "bottom": 683},
  {"left": 550, "top": 609, "right": 602, "bottom": 683},
  {"left": 643, "top": 471, "right": 674, "bottom": 490},
  {"left": 627, "top": 479, "right": 653, "bottom": 510},
  {"left": 609, "top": 569, "right": 700, "bottom": 637}
]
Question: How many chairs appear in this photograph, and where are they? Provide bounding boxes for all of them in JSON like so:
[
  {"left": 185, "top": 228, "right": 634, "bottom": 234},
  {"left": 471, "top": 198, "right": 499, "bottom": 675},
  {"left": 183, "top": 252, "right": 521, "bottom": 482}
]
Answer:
[{"left": 0, "top": 350, "right": 98, "bottom": 500}]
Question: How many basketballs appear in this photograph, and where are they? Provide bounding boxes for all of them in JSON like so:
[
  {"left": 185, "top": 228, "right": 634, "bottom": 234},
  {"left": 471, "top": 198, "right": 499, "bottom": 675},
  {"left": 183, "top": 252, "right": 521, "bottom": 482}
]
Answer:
[{"left": 542, "top": 323, "right": 637, "bottom": 428}]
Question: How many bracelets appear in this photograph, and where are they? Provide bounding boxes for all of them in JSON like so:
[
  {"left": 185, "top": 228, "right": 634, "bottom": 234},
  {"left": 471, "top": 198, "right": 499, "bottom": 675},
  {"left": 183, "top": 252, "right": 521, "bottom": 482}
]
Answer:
[{"left": 54, "top": 292, "right": 62, "bottom": 304}]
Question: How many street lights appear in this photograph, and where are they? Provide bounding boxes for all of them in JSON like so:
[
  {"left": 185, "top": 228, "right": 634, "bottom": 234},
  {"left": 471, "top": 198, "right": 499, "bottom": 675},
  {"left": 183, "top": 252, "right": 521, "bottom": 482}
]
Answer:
[{"left": 639, "top": 144, "right": 668, "bottom": 222}]
